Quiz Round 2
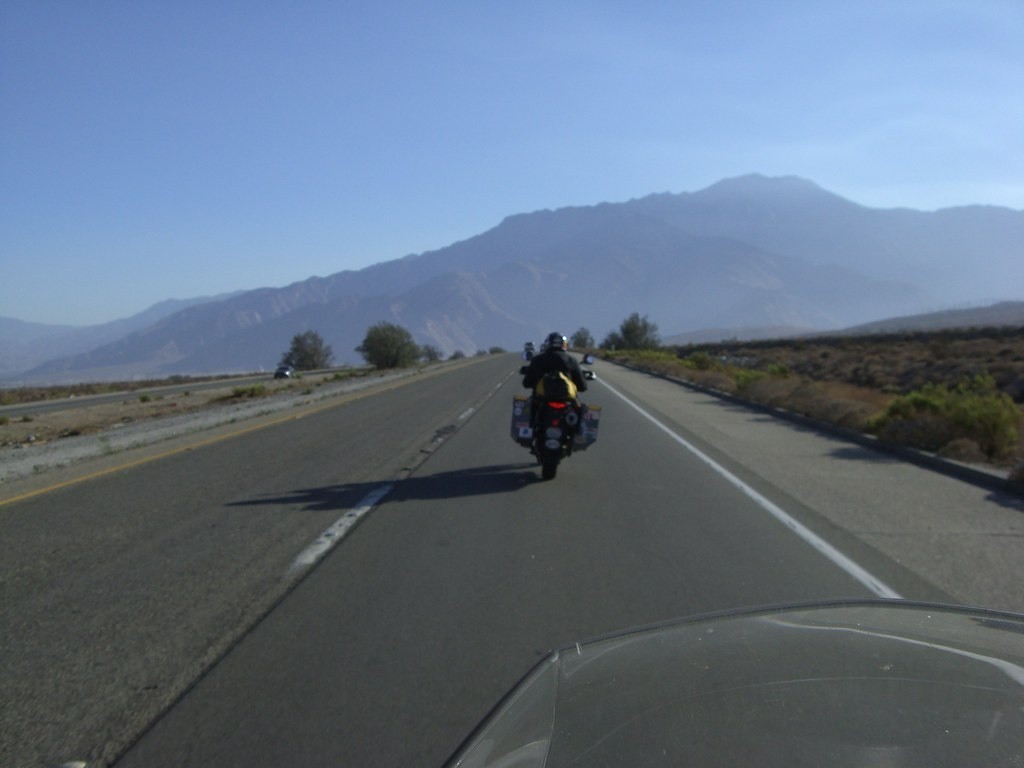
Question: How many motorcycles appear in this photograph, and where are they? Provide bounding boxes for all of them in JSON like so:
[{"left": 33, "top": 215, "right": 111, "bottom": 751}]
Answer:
[{"left": 509, "top": 348, "right": 602, "bottom": 482}]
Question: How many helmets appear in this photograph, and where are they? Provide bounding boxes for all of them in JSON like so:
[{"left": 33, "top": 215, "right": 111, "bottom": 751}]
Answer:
[{"left": 544, "top": 332, "right": 567, "bottom": 352}]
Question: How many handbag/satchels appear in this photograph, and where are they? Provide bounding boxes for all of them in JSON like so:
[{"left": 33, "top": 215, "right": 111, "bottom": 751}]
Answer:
[{"left": 535, "top": 371, "right": 577, "bottom": 400}]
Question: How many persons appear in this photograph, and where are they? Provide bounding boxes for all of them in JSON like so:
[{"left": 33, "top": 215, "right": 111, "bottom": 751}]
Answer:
[{"left": 522, "top": 332, "right": 588, "bottom": 450}]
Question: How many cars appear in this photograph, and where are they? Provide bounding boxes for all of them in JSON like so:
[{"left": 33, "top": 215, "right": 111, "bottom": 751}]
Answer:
[
  {"left": 538, "top": 335, "right": 567, "bottom": 351},
  {"left": 272, "top": 365, "right": 294, "bottom": 379},
  {"left": 523, "top": 342, "right": 535, "bottom": 351}
]
